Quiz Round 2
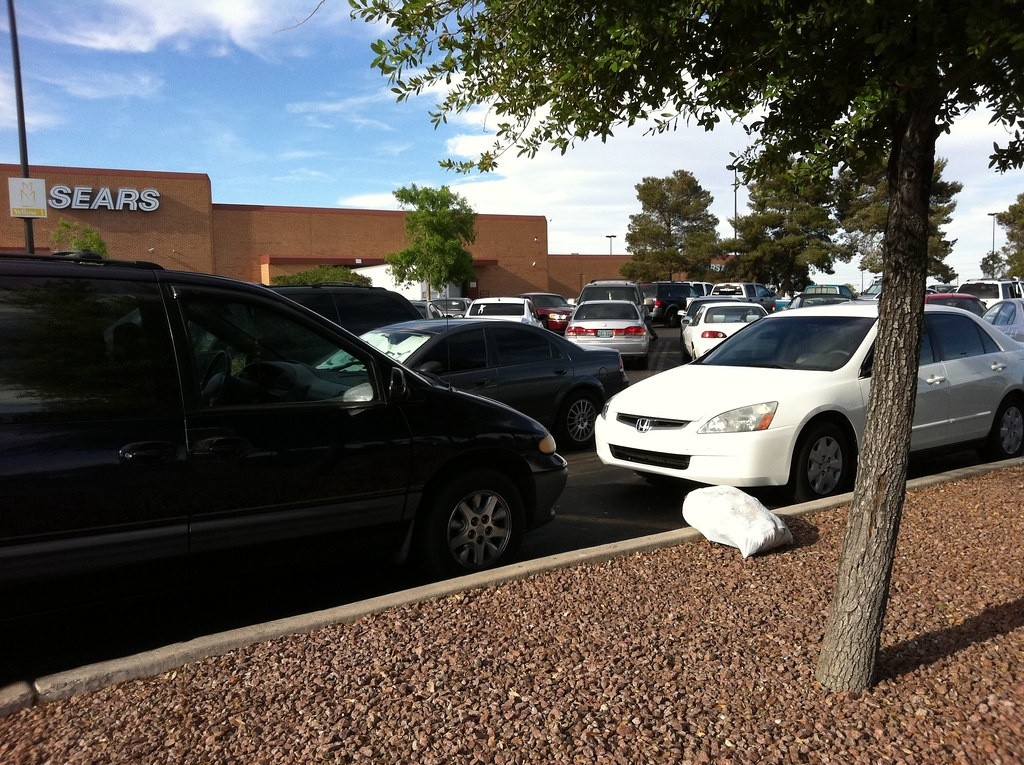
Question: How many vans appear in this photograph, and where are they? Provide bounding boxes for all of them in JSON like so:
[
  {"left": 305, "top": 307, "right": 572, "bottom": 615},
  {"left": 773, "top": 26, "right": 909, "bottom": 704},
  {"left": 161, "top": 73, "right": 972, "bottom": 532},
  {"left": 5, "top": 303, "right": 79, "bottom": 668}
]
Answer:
[{"left": 2, "top": 251, "right": 568, "bottom": 592}]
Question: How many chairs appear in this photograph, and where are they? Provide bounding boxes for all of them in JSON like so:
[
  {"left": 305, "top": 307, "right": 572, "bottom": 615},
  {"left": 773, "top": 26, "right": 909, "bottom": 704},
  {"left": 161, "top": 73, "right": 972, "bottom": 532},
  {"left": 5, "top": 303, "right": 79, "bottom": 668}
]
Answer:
[
  {"left": 712, "top": 314, "right": 726, "bottom": 322},
  {"left": 795, "top": 330, "right": 841, "bottom": 367},
  {"left": 106, "top": 323, "right": 145, "bottom": 379},
  {"left": 619, "top": 311, "right": 633, "bottom": 318},
  {"left": 746, "top": 315, "right": 760, "bottom": 322}
]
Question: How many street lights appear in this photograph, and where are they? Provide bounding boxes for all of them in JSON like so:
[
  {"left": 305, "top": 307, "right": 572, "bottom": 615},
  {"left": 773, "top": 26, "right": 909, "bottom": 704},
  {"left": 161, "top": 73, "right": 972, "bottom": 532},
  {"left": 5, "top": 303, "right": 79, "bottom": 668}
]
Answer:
[{"left": 988, "top": 212, "right": 997, "bottom": 280}]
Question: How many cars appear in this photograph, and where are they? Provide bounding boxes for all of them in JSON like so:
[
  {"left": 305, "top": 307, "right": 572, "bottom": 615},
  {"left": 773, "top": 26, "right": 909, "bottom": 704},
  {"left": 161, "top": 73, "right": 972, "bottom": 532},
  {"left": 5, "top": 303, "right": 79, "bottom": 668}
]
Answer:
[
  {"left": 252, "top": 282, "right": 628, "bottom": 451},
  {"left": 408, "top": 277, "right": 1024, "bottom": 367},
  {"left": 594, "top": 305, "right": 1024, "bottom": 500}
]
